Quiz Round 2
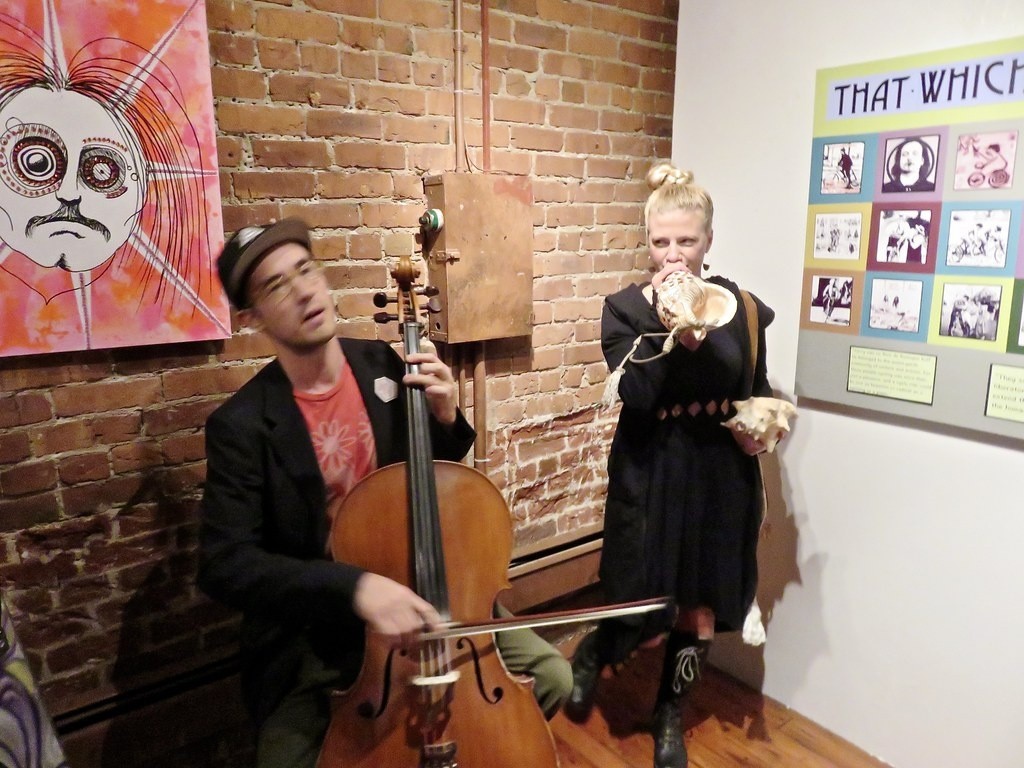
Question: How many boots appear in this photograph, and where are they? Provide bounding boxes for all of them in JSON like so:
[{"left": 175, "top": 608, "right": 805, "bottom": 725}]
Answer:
[
  {"left": 654, "top": 627, "right": 712, "bottom": 768},
  {"left": 565, "top": 628, "right": 622, "bottom": 722}
]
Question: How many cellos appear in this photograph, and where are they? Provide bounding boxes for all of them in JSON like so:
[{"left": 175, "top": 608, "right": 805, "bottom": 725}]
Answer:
[{"left": 312, "top": 256, "right": 560, "bottom": 768}]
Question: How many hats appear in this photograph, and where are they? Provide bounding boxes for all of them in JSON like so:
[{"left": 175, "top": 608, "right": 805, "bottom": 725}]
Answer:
[{"left": 216, "top": 217, "right": 310, "bottom": 310}]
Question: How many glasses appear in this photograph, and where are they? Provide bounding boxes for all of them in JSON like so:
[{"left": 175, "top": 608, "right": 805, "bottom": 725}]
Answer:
[{"left": 249, "top": 264, "right": 327, "bottom": 304}]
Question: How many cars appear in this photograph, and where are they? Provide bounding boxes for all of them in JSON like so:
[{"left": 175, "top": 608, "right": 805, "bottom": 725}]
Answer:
[{"left": 960, "top": 286, "right": 1004, "bottom": 340}]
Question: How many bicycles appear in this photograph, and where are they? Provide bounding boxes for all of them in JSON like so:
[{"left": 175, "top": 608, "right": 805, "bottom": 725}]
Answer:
[
  {"left": 823, "top": 162, "right": 862, "bottom": 191},
  {"left": 950, "top": 224, "right": 1008, "bottom": 264},
  {"left": 821, "top": 293, "right": 837, "bottom": 323}
]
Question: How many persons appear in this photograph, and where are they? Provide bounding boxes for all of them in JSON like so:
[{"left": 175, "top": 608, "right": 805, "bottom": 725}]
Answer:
[
  {"left": 562, "top": 164, "right": 788, "bottom": 768},
  {"left": 198, "top": 216, "right": 573, "bottom": 768}
]
